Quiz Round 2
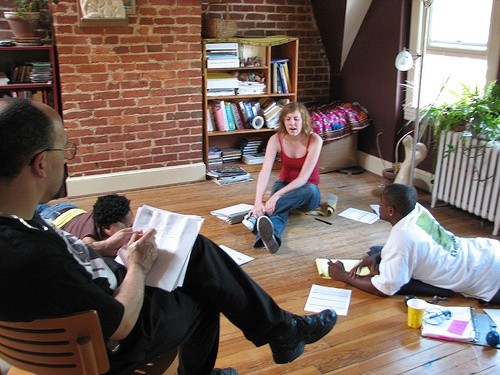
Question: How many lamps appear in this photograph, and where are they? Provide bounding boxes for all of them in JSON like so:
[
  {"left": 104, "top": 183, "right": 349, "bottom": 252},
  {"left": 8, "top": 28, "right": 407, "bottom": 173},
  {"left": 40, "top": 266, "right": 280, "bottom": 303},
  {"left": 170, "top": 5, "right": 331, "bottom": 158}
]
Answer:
[{"left": 395, "top": 0, "right": 434, "bottom": 188}]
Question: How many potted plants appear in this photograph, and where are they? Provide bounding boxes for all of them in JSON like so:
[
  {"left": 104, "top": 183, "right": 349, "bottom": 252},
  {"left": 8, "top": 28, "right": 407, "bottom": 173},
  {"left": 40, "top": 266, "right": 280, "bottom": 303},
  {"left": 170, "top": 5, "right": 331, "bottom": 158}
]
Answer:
[
  {"left": 419, "top": 81, "right": 500, "bottom": 158},
  {"left": 3, "top": 0, "right": 60, "bottom": 39},
  {"left": 383, "top": 163, "right": 400, "bottom": 187}
]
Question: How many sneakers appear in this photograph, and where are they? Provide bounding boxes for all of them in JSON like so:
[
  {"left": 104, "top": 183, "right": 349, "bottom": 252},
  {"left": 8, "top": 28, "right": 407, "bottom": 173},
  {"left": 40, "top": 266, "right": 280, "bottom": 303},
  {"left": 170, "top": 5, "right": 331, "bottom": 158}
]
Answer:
[
  {"left": 257, "top": 215, "right": 280, "bottom": 254},
  {"left": 242, "top": 214, "right": 257, "bottom": 232},
  {"left": 180, "top": 367, "right": 237, "bottom": 375},
  {"left": 269, "top": 309, "right": 338, "bottom": 365}
]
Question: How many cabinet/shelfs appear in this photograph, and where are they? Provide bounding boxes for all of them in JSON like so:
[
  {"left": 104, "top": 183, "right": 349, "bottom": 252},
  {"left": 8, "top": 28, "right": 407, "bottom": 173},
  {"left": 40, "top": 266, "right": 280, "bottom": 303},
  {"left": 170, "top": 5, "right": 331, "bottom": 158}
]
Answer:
[
  {"left": 0, "top": 44, "right": 65, "bottom": 198},
  {"left": 202, "top": 38, "right": 299, "bottom": 169}
]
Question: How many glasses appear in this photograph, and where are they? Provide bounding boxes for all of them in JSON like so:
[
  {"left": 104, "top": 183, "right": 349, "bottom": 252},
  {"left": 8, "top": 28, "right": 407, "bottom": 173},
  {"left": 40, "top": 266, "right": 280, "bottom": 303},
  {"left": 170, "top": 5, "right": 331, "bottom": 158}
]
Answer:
[{"left": 28, "top": 142, "right": 79, "bottom": 167}]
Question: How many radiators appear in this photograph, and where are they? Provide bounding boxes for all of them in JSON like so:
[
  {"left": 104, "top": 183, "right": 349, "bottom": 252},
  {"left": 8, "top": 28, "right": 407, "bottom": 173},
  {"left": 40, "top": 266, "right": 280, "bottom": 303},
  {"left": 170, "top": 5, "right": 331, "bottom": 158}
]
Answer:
[{"left": 432, "top": 129, "right": 500, "bottom": 236}]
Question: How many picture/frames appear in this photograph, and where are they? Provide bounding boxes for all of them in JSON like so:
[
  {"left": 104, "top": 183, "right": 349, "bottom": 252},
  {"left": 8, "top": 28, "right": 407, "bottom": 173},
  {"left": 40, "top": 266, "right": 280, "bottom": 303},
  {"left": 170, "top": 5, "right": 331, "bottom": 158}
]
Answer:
[{"left": 123, "top": 0, "right": 135, "bottom": 14}]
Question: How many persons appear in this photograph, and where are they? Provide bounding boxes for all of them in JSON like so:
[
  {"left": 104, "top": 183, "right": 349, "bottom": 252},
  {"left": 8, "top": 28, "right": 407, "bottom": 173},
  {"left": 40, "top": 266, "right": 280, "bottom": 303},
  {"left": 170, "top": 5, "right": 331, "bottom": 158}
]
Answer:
[
  {"left": 0, "top": 96, "right": 338, "bottom": 374},
  {"left": 35, "top": 192, "right": 136, "bottom": 245},
  {"left": 241, "top": 101, "right": 323, "bottom": 255},
  {"left": 327, "top": 184, "right": 500, "bottom": 306}
]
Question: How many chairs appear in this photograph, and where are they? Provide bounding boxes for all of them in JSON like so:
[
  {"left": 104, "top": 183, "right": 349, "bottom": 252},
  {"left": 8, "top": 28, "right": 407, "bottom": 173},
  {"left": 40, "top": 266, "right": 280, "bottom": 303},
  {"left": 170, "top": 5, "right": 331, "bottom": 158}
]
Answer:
[{"left": 0, "top": 309, "right": 178, "bottom": 375}]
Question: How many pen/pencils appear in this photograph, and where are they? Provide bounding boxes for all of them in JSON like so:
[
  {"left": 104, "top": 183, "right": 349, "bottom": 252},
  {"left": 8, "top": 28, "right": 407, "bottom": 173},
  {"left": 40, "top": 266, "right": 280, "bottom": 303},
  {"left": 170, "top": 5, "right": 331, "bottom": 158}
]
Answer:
[
  {"left": 328, "top": 258, "right": 332, "bottom": 263},
  {"left": 315, "top": 218, "right": 332, "bottom": 225}
]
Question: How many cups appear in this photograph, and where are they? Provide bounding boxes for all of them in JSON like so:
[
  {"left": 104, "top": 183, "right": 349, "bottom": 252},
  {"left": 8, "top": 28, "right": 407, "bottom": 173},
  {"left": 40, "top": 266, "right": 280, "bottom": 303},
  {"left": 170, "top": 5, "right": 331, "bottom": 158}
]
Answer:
[{"left": 406, "top": 298, "right": 428, "bottom": 328}]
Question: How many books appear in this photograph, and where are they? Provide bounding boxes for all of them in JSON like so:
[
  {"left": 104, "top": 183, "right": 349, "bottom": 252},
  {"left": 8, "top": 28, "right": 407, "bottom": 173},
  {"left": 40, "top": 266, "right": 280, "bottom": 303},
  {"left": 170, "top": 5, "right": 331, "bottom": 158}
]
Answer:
[
  {"left": 0, "top": 59, "right": 56, "bottom": 108},
  {"left": 205, "top": 43, "right": 292, "bottom": 186}
]
